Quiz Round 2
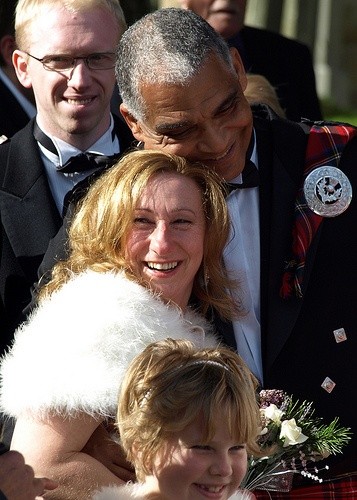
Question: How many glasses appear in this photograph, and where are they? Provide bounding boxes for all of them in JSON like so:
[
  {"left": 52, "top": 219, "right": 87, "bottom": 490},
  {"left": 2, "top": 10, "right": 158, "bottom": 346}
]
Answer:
[{"left": 18, "top": 48, "right": 119, "bottom": 72}]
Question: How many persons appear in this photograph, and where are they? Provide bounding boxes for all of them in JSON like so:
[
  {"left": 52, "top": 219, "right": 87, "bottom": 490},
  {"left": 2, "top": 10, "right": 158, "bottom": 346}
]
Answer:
[
  {"left": 89, "top": 335, "right": 258, "bottom": 500},
  {"left": 0, "top": 0, "right": 357, "bottom": 500}
]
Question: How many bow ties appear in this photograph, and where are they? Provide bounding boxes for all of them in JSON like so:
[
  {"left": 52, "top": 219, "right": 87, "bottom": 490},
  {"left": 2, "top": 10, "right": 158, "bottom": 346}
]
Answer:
[
  {"left": 218, "top": 127, "right": 260, "bottom": 199},
  {"left": 31, "top": 118, "right": 121, "bottom": 178}
]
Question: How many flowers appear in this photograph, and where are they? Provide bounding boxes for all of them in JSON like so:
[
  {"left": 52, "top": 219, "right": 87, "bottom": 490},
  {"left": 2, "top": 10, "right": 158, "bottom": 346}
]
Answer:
[{"left": 238, "top": 389, "right": 355, "bottom": 500}]
